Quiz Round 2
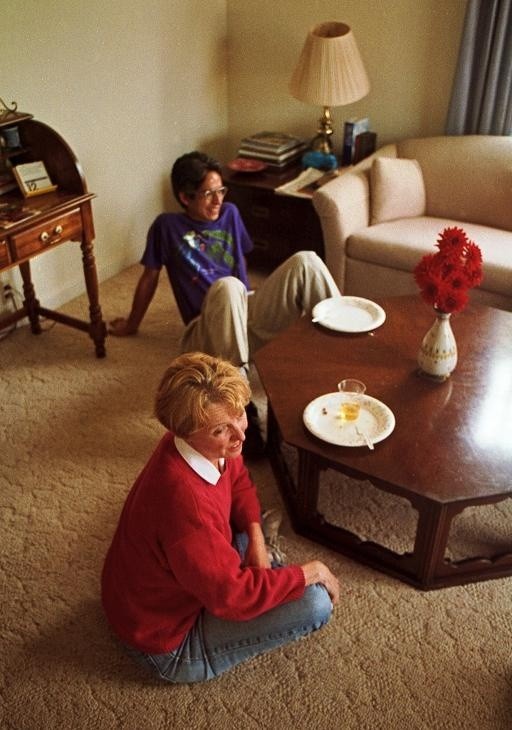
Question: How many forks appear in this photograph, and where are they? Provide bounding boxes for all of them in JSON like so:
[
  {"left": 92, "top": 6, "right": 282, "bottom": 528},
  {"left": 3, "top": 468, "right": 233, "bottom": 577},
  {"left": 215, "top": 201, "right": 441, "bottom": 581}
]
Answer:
[{"left": 356, "top": 422, "right": 375, "bottom": 451}]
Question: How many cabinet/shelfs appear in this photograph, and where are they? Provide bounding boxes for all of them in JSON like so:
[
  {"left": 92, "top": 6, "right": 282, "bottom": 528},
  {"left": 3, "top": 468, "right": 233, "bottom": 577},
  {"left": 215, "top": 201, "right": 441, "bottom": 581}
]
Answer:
[
  {"left": 0, "top": 96, "right": 107, "bottom": 358},
  {"left": 219, "top": 162, "right": 353, "bottom": 274}
]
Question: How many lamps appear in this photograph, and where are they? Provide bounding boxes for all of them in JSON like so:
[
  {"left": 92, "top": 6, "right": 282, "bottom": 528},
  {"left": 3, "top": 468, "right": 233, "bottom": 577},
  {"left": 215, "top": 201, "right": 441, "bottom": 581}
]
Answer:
[{"left": 289, "top": 22, "right": 371, "bottom": 153}]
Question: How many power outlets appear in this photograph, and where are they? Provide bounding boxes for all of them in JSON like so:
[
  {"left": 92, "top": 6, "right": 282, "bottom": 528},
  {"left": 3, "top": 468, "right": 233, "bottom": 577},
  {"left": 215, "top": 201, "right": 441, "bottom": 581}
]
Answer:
[{"left": 0, "top": 279, "right": 16, "bottom": 303}]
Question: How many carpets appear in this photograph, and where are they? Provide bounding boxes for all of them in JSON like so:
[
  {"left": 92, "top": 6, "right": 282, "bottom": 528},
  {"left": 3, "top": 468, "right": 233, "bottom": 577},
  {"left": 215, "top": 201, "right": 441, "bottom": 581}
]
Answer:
[{"left": 0, "top": 262, "right": 512, "bottom": 730}]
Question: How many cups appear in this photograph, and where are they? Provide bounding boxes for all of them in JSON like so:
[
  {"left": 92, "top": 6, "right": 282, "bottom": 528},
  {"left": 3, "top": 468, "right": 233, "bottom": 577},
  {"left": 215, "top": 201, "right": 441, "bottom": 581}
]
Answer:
[{"left": 337, "top": 378, "right": 367, "bottom": 420}]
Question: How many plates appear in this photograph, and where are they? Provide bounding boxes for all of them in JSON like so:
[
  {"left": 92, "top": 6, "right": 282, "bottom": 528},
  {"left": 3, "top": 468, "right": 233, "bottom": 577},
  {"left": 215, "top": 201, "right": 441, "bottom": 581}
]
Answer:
[
  {"left": 311, "top": 296, "right": 386, "bottom": 334},
  {"left": 226, "top": 157, "right": 268, "bottom": 173},
  {"left": 302, "top": 390, "right": 397, "bottom": 448}
]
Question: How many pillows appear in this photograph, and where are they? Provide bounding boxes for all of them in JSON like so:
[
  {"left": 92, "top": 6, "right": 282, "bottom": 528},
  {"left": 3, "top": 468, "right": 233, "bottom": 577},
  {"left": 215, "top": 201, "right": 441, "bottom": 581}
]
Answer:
[{"left": 370, "top": 157, "right": 425, "bottom": 225}]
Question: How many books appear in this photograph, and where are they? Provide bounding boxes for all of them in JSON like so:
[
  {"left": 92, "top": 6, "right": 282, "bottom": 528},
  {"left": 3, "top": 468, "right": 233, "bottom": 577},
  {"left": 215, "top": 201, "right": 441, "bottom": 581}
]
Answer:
[
  {"left": 344, "top": 116, "right": 376, "bottom": 164},
  {"left": 235, "top": 130, "right": 310, "bottom": 169}
]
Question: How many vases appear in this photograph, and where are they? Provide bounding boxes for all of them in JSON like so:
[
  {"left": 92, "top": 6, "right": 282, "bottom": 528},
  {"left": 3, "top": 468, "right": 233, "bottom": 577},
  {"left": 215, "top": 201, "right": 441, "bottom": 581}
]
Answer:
[{"left": 419, "top": 313, "right": 456, "bottom": 383}]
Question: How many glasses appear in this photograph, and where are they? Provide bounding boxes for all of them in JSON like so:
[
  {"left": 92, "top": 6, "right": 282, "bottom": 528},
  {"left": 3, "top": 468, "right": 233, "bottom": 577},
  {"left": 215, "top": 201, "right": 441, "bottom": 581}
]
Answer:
[{"left": 186, "top": 187, "right": 229, "bottom": 200}]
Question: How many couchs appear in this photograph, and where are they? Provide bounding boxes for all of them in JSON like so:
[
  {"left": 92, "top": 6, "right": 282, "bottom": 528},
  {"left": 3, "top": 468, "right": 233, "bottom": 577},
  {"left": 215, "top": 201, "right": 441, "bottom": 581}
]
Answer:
[{"left": 312, "top": 135, "right": 512, "bottom": 311}]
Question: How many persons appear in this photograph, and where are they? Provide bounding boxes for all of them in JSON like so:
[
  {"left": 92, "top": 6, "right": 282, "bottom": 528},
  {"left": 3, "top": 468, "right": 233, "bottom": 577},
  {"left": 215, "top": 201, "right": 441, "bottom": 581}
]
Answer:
[
  {"left": 101, "top": 353, "right": 339, "bottom": 684},
  {"left": 106, "top": 149, "right": 344, "bottom": 452}
]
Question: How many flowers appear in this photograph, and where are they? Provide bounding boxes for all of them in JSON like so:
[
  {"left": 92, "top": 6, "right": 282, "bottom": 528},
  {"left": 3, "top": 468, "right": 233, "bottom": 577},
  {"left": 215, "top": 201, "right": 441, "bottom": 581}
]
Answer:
[{"left": 414, "top": 226, "right": 485, "bottom": 313}]
